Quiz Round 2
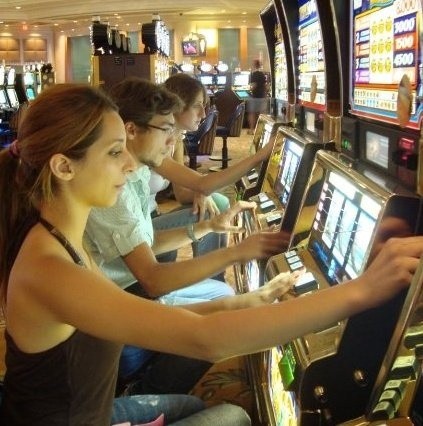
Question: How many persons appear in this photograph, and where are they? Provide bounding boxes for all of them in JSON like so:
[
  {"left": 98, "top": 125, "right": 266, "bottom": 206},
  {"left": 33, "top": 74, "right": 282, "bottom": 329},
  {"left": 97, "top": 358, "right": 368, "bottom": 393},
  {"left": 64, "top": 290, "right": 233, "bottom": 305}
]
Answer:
[
  {"left": 150, "top": 73, "right": 276, "bottom": 284},
  {"left": 0, "top": 82, "right": 423, "bottom": 426},
  {"left": 248, "top": 60, "right": 267, "bottom": 135},
  {"left": 83, "top": 77, "right": 291, "bottom": 395}
]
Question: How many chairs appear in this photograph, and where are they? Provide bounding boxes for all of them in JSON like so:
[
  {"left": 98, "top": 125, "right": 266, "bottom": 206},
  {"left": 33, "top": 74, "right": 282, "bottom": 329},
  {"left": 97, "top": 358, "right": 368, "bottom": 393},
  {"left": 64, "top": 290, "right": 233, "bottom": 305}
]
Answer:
[
  {"left": 209, "top": 100, "right": 246, "bottom": 172},
  {"left": 180, "top": 110, "right": 218, "bottom": 171}
]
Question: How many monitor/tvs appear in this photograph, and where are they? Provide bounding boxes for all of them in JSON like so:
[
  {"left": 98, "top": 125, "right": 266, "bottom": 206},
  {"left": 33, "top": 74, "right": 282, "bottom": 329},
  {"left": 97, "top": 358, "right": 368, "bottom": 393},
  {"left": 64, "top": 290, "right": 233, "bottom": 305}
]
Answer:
[
  {"left": 199, "top": 72, "right": 249, "bottom": 86},
  {"left": 182, "top": 40, "right": 200, "bottom": 57},
  {"left": 0, "top": 68, "right": 39, "bottom": 110},
  {"left": 273, "top": 138, "right": 302, "bottom": 204},
  {"left": 248, "top": 117, "right": 271, "bottom": 172},
  {"left": 304, "top": 168, "right": 383, "bottom": 287}
]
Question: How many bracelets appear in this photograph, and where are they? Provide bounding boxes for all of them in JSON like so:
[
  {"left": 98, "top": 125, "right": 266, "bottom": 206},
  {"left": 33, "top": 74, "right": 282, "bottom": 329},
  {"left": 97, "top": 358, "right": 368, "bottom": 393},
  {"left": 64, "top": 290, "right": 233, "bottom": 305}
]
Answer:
[{"left": 188, "top": 224, "right": 203, "bottom": 243}]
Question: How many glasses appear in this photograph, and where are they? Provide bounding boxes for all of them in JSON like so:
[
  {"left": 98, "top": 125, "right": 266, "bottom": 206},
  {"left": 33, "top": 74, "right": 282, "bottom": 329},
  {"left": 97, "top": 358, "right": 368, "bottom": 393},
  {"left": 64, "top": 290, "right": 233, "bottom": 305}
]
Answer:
[{"left": 147, "top": 124, "right": 173, "bottom": 135}]
